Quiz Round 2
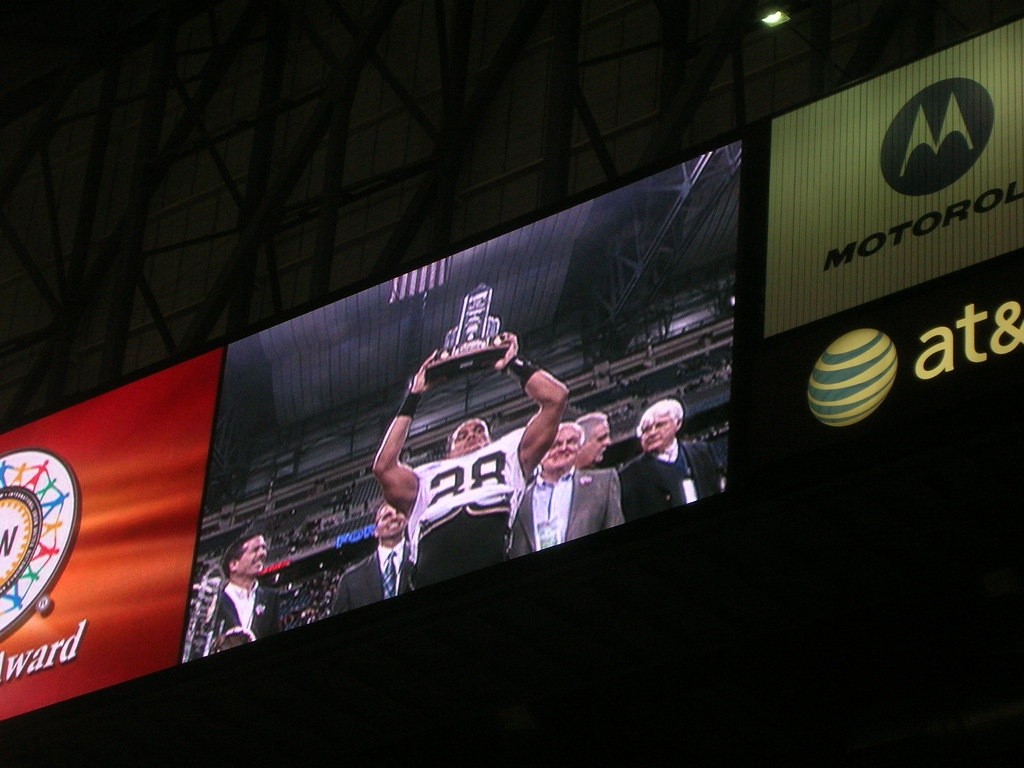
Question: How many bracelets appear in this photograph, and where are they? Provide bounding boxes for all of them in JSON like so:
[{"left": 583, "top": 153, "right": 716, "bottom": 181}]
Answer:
[
  {"left": 502, "top": 354, "right": 540, "bottom": 391},
  {"left": 396, "top": 393, "right": 421, "bottom": 420}
]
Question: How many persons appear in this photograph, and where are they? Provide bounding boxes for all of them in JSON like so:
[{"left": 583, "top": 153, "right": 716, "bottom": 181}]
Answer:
[{"left": 208, "top": 329, "right": 726, "bottom": 656}]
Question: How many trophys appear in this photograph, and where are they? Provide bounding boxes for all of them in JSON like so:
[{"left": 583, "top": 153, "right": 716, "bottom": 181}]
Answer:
[{"left": 423, "top": 281, "right": 509, "bottom": 388}]
